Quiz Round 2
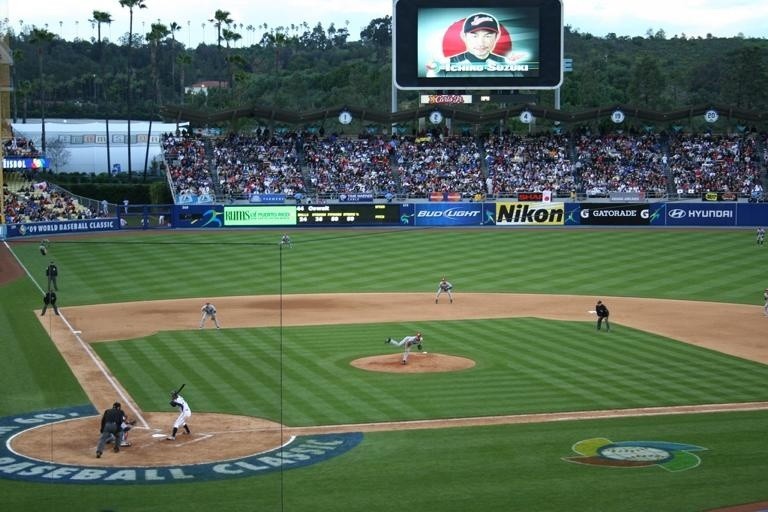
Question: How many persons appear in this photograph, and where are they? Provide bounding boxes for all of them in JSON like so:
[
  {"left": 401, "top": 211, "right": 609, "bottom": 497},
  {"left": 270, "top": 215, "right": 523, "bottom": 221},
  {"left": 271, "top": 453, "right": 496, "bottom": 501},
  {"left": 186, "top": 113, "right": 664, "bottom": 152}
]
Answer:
[
  {"left": 426, "top": 12, "right": 524, "bottom": 77},
  {"left": 0, "top": 137, "right": 42, "bottom": 156},
  {"left": 199, "top": 302, "right": 220, "bottom": 330},
  {"left": 757, "top": 225, "right": 765, "bottom": 245},
  {"left": 595, "top": 300, "right": 610, "bottom": 332},
  {"left": 105, "top": 410, "right": 137, "bottom": 447},
  {"left": 0, "top": 171, "right": 108, "bottom": 223},
  {"left": 385, "top": 331, "right": 423, "bottom": 365},
  {"left": 162, "top": 119, "right": 767, "bottom": 202},
  {"left": 434, "top": 277, "right": 453, "bottom": 304},
  {"left": 165, "top": 389, "right": 191, "bottom": 440},
  {"left": 282, "top": 233, "right": 292, "bottom": 248},
  {"left": 120, "top": 208, "right": 165, "bottom": 226},
  {"left": 40, "top": 239, "right": 50, "bottom": 254},
  {"left": 763, "top": 288, "right": 768, "bottom": 316},
  {"left": 40, "top": 288, "right": 59, "bottom": 316},
  {"left": 46, "top": 261, "right": 58, "bottom": 291},
  {"left": 96, "top": 402, "right": 123, "bottom": 458}
]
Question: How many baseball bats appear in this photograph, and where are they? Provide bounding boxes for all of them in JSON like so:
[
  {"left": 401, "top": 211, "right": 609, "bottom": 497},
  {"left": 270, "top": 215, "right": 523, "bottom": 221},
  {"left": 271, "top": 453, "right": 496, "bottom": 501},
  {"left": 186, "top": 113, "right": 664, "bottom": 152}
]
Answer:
[{"left": 177, "top": 385, "right": 185, "bottom": 395}]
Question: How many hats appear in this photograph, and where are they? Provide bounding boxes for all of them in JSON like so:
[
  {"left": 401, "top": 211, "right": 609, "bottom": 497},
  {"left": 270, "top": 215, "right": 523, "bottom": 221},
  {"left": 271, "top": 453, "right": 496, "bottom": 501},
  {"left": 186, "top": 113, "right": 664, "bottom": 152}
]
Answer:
[{"left": 463, "top": 11, "right": 500, "bottom": 35}]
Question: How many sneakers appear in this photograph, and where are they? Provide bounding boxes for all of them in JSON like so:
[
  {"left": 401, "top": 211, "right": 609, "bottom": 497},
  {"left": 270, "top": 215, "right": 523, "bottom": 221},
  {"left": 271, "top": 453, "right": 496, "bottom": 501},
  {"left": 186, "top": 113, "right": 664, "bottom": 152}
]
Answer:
[
  {"left": 95, "top": 430, "right": 192, "bottom": 459},
  {"left": 383, "top": 337, "right": 407, "bottom": 367}
]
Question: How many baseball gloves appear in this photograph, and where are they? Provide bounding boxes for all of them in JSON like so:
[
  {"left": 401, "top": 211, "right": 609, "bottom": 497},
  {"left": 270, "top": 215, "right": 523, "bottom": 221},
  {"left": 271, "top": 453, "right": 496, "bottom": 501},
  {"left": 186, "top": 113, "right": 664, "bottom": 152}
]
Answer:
[
  {"left": 127, "top": 419, "right": 136, "bottom": 426},
  {"left": 418, "top": 344, "right": 422, "bottom": 350}
]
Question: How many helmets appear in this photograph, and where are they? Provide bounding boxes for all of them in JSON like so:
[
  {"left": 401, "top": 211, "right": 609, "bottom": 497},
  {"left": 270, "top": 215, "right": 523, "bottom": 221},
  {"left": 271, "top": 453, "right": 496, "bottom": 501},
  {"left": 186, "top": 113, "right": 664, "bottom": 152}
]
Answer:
[{"left": 114, "top": 391, "right": 180, "bottom": 411}]
